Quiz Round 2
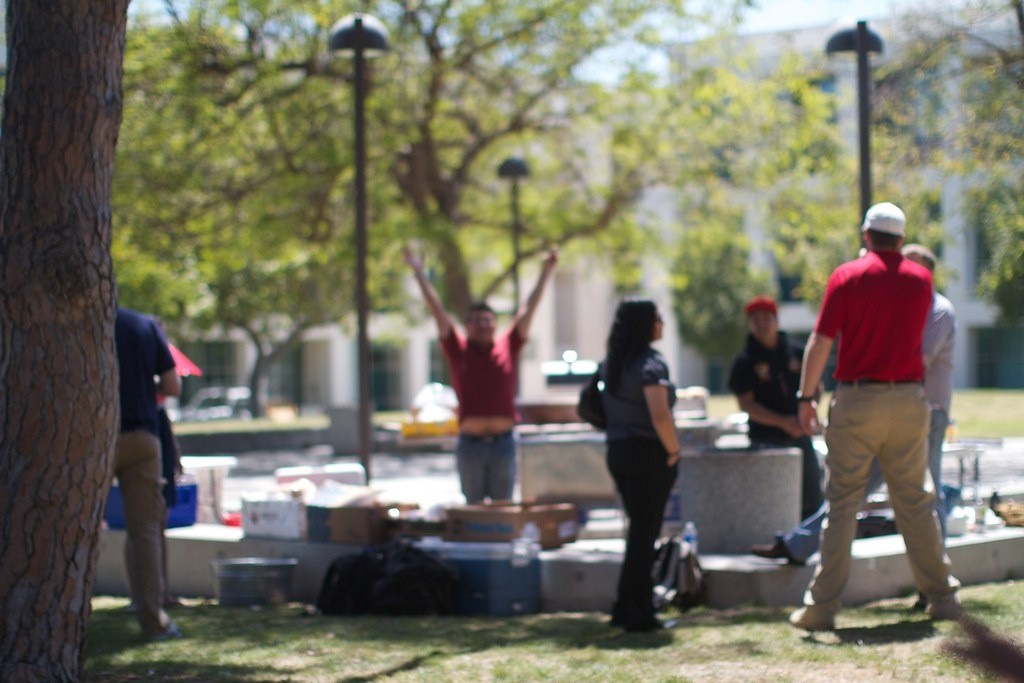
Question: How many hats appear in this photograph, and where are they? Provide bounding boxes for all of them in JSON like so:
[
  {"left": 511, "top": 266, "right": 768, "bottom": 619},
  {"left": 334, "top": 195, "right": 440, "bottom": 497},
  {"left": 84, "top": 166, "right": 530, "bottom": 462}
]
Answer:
[
  {"left": 862, "top": 201, "right": 907, "bottom": 236},
  {"left": 745, "top": 295, "right": 777, "bottom": 314}
]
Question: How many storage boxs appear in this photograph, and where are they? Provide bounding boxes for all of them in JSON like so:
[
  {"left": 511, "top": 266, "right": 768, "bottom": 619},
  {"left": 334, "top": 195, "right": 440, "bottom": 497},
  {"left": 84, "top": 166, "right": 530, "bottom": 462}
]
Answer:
[
  {"left": 213, "top": 556, "right": 301, "bottom": 607},
  {"left": 172, "top": 456, "right": 238, "bottom": 526},
  {"left": 239, "top": 489, "right": 306, "bottom": 540},
  {"left": 443, "top": 499, "right": 580, "bottom": 548},
  {"left": 105, "top": 481, "right": 198, "bottom": 530},
  {"left": 304, "top": 486, "right": 419, "bottom": 538}
]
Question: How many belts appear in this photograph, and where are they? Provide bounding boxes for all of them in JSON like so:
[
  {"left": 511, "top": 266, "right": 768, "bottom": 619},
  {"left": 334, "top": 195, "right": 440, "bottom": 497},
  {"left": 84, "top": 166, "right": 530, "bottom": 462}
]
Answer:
[{"left": 840, "top": 377, "right": 921, "bottom": 386}]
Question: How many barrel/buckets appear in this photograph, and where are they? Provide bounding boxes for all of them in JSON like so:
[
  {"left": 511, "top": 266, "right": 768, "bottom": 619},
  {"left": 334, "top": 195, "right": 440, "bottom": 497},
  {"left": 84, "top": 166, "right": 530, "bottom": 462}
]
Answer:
[{"left": 211, "top": 559, "right": 298, "bottom": 605}]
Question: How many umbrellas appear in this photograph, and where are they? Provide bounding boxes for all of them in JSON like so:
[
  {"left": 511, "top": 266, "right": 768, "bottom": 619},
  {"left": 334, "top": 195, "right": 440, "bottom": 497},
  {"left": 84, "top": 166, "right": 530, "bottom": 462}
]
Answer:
[{"left": 154, "top": 343, "right": 202, "bottom": 377}]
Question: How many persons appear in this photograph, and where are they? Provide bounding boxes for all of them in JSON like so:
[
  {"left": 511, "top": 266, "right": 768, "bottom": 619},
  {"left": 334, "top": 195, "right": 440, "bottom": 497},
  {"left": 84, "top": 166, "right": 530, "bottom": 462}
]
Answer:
[
  {"left": 403, "top": 243, "right": 559, "bottom": 505},
  {"left": 729, "top": 244, "right": 957, "bottom": 611},
  {"left": 113, "top": 305, "right": 185, "bottom": 637},
  {"left": 602, "top": 299, "right": 681, "bottom": 633},
  {"left": 789, "top": 201, "right": 961, "bottom": 629}
]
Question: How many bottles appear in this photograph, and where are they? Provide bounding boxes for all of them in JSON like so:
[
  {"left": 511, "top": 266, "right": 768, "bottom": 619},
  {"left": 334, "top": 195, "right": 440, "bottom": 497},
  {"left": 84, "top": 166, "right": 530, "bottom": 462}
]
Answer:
[
  {"left": 959, "top": 457, "right": 1001, "bottom": 531},
  {"left": 513, "top": 524, "right": 540, "bottom": 567},
  {"left": 680, "top": 523, "right": 697, "bottom": 558}
]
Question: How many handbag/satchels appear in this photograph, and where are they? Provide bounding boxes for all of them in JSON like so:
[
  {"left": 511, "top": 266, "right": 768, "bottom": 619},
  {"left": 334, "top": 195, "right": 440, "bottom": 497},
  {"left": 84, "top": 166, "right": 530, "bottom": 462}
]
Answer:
[
  {"left": 319, "top": 543, "right": 450, "bottom": 616},
  {"left": 576, "top": 363, "right": 610, "bottom": 429}
]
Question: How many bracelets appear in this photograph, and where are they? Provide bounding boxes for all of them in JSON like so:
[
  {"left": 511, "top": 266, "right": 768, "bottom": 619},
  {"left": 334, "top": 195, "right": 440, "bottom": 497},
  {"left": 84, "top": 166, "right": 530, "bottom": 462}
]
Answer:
[
  {"left": 668, "top": 447, "right": 682, "bottom": 456},
  {"left": 795, "top": 390, "right": 814, "bottom": 403}
]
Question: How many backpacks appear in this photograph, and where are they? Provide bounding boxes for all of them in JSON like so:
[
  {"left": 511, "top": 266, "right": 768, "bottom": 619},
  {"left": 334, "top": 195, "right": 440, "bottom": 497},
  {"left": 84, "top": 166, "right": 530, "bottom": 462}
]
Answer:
[{"left": 653, "top": 536, "right": 703, "bottom": 609}]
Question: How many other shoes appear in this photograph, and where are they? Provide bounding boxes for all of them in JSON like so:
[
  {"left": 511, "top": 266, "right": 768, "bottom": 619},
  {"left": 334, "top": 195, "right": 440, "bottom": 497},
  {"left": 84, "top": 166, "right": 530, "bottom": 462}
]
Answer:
[
  {"left": 611, "top": 605, "right": 676, "bottom": 632},
  {"left": 751, "top": 542, "right": 807, "bottom": 565},
  {"left": 926, "top": 594, "right": 959, "bottom": 614},
  {"left": 154, "top": 620, "right": 183, "bottom": 638},
  {"left": 790, "top": 606, "right": 836, "bottom": 630}
]
